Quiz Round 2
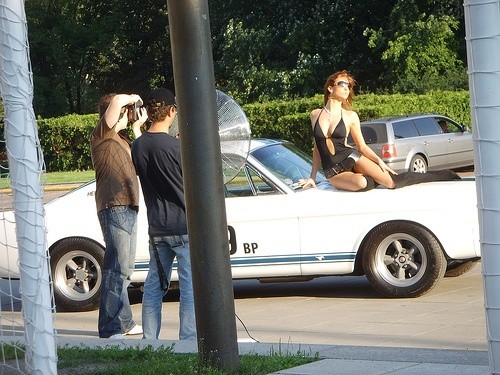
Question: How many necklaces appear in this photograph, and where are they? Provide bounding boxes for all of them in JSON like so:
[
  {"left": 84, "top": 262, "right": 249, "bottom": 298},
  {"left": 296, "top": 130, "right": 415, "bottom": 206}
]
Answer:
[{"left": 324, "top": 107, "right": 341, "bottom": 115}]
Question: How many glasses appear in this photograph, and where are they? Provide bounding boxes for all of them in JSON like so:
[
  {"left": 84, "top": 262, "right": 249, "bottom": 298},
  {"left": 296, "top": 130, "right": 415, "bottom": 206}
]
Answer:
[{"left": 333, "top": 81, "right": 352, "bottom": 89}]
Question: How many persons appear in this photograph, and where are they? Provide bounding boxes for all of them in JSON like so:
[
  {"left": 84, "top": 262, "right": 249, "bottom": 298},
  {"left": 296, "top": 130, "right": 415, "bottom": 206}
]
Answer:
[
  {"left": 90, "top": 93, "right": 148, "bottom": 339},
  {"left": 439, "top": 121, "right": 449, "bottom": 132},
  {"left": 131, "top": 88, "right": 198, "bottom": 341},
  {"left": 298, "top": 70, "right": 461, "bottom": 192}
]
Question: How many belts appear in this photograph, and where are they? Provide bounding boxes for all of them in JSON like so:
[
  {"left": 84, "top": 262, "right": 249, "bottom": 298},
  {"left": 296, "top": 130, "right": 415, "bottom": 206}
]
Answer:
[{"left": 324, "top": 150, "right": 363, "bottom": 179}]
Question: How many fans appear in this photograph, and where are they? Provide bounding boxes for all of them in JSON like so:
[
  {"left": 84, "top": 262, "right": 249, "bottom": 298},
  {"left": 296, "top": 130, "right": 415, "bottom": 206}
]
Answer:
[{"left": 168, "top": 90, "right": 251, "bottom": 184}]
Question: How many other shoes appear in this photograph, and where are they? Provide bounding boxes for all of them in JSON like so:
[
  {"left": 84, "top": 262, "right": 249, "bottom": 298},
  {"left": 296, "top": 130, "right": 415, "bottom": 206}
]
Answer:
[
  {"left": 124, "top": 324, "right": 143, "bottom": 334},
  {"left": 109, "top": 334, "right": 126, "bottom": 339}
]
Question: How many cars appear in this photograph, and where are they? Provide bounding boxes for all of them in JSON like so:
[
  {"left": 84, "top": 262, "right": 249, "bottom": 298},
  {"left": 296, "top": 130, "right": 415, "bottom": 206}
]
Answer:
[{"left": 0, "top": 87, "right": 481, "bottom": 312}]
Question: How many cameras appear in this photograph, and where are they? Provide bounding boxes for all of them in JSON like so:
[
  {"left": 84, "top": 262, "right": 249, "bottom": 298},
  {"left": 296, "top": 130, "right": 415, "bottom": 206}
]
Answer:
[{"left": 132, "top": 100, "right": 143, "bottom": 121}]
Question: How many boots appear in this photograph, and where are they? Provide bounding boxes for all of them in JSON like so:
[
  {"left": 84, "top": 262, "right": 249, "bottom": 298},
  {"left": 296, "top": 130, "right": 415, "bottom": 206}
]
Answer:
[
  {"left": 388, "top": 170, "right": 462, "bottom": 189},
  {"left": 356, "top": 174, "right": 381, "bottom": 192}
]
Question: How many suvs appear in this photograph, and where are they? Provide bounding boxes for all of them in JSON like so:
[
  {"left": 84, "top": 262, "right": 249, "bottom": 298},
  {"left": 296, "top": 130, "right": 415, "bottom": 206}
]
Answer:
[{"left": 346, "top": 112, "right": 474, "bottom": 175}]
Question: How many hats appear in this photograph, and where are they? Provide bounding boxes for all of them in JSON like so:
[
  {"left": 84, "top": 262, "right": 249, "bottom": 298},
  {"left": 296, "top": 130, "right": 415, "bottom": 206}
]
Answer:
[{"left": 145, "top": 87, "right": 176, "bottom": 107}]
